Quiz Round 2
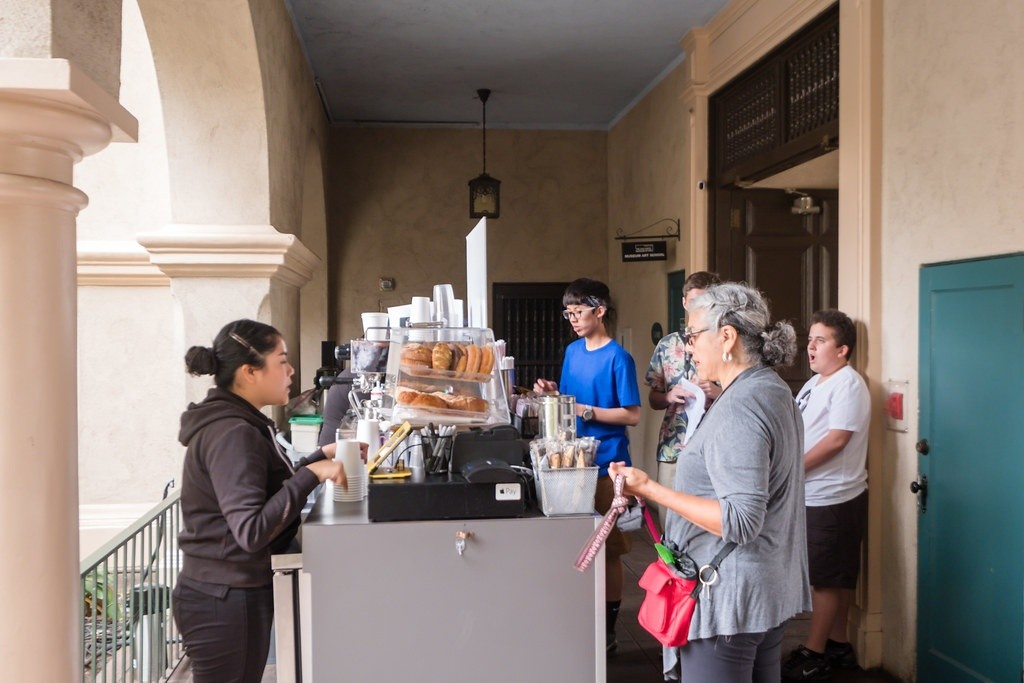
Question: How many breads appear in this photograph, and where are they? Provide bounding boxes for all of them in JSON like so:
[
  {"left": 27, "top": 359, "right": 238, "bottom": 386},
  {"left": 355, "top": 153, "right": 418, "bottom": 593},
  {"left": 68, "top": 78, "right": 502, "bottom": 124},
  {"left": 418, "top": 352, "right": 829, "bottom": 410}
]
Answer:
[
  {"left": 396, "top": 391, "right": 489, "bottom": 416},
  {"left": 399, "top": 343, "right": 494, "bottom": 381}
]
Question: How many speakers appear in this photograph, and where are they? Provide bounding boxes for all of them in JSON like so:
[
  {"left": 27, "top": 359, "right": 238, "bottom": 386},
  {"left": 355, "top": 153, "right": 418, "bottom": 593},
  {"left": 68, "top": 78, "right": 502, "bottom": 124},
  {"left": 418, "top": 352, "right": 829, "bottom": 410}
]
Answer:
[{"left": 321, "top": 341, "right": 336, "bottom": 369}]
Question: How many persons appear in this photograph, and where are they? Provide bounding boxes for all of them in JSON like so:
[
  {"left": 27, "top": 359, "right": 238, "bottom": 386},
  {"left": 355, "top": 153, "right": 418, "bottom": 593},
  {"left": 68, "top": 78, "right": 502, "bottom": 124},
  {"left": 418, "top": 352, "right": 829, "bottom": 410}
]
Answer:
[
  {"left": 781, "top": 308, "right": 872, "bottom": 683},
  {"left": 171, "top": 317, "right": 388, "bottom": 683},
  {"left": 607, "top": 280, "right": 813, "bottom": 683},
  {"left": 643, "top": 271, "right": 722, "bottom": 683},
  {"left": 533, "top": 277, "right": 642, "bottom": 652}
]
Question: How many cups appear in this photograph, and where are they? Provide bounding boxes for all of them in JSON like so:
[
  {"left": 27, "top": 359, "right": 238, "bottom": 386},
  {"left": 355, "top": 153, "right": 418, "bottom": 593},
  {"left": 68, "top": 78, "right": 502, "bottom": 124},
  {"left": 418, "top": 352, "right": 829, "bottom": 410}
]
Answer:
[
  {"left": 433, "top": 284, "right": 456, "bottom": 341},
  {"left": 408, "top": 296, "right": 434, "bottom": 342},
  {"left": 537, "top": 394, "right": 559, "bottom": 441},
  {"left": 332, "top": 428, "right": 364, "bottom": 502},
  {"left": 409, "top": 434, "right": 426, "bottom": 483},
  {"left": 558, "top": 395, "right": 576, "bottom": 442}
]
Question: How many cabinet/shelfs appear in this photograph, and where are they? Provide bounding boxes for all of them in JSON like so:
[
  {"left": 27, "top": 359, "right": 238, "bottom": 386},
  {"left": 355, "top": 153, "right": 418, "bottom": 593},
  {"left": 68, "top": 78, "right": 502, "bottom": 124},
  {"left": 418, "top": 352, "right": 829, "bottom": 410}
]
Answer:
[{"left": 378, "top": 327, "right": 511, "bottom": 427}]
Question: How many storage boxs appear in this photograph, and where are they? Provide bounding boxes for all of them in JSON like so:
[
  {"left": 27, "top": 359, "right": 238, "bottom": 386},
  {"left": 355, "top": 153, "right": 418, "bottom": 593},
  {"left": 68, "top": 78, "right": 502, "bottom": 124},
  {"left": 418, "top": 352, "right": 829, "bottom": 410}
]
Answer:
[{"left": 529, "top": 459, "right": 600, "bottom": 517}]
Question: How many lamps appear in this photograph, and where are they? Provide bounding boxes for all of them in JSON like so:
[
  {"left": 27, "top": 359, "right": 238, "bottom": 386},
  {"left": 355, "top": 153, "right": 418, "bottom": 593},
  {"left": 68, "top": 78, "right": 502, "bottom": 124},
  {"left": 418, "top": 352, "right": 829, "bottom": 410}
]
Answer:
[{"left": 467, "top": 89, "right": 502, "bottom": 220}]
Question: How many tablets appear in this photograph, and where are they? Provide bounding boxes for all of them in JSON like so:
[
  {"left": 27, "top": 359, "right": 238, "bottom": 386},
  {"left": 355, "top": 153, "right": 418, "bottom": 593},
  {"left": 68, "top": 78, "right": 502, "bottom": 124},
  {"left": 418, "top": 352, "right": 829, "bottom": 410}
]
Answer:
[{"left": 367, "top": 421, "right": 413, "bottom": 475}]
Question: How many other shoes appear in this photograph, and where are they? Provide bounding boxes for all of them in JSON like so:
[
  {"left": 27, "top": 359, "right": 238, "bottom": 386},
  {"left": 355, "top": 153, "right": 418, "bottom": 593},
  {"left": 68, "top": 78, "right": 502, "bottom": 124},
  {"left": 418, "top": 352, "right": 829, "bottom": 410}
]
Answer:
[{"left": 605, "top": 633, "right": 620, "bottom": 655}]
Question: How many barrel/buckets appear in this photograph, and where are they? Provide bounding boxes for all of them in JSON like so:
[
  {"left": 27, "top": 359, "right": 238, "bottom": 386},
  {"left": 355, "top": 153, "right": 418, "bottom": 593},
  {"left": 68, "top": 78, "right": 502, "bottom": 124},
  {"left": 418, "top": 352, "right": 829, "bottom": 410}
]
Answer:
[{"left": 360, "top": 312, "right": 389, "bottom": 340}]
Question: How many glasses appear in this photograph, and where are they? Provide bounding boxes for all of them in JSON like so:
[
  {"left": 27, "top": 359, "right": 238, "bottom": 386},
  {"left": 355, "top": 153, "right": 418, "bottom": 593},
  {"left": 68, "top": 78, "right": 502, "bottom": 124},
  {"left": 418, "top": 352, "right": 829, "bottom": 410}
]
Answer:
[
  {"left": 683, "top": 328, "right": 712, "bottom": 344},
  {"left": 562, "top": 306, "right": 596, "bottom": 321}
]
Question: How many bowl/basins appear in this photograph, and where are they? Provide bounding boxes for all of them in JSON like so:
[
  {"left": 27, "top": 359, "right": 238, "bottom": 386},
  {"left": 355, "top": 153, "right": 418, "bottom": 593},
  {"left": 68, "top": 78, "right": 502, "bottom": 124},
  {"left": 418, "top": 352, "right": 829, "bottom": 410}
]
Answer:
[{"left": 357, "top": 419, "right": 382, "bottom": 465}]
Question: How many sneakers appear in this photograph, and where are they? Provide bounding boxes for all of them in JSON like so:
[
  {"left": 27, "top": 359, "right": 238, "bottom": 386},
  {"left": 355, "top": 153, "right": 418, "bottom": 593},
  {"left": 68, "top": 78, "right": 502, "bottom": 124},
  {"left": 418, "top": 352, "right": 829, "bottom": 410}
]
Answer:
[
  {"left": 824, "top": 638, "right": 860, "bottom": 679},
  {"left": 781, "top": 643, "right": 834, "bottom": 683}
]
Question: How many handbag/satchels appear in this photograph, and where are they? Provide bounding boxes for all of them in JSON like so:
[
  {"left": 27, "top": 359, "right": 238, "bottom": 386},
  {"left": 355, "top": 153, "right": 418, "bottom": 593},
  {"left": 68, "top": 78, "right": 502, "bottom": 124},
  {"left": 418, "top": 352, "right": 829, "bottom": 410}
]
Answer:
[{"left": 637, "top": 556, "right": 702, "bottom": 651}]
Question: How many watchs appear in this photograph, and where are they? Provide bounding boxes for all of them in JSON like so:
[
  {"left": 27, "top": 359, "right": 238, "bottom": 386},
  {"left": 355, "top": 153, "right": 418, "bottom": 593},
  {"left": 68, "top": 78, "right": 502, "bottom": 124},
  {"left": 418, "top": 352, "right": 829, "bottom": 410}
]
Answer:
[{"left": 583, "top": 404, "right": 593, "bottom": 421}]
